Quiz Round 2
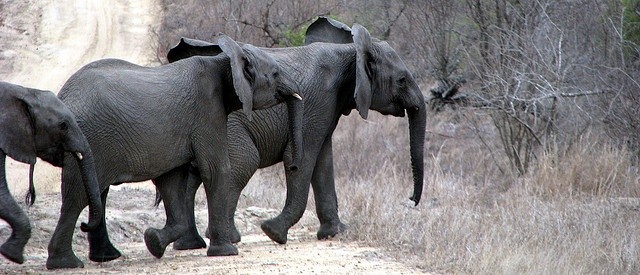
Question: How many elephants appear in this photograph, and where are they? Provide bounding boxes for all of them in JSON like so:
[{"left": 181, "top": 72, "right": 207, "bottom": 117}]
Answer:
[
  {"left": 0, "top": 81, "right": 103, "bottom": 264},
  {"left": 25, "top": 31, "right": 304, "bottom": 271},
  {"left": 172, "top": 15, "right": 427, "bottom": 257}
]
